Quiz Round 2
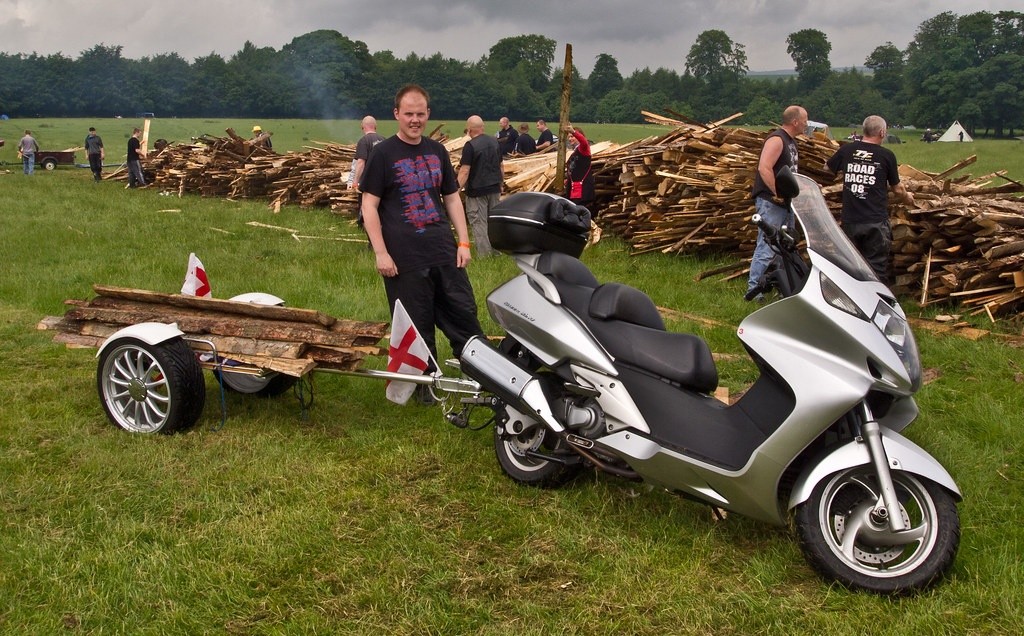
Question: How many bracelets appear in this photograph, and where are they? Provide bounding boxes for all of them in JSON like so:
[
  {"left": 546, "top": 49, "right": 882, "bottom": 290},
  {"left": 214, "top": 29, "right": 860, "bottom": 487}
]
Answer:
[{"left": 458, "top": 242, "right": 469, "bottom": 248}]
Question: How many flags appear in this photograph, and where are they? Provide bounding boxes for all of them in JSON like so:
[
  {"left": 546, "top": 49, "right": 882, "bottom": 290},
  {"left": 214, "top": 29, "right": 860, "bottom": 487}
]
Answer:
[
  {"left": 181, "top": 253, "right": 212, "bottom": 298},
  {"left": 386, "top": 299, "right": 429, "bottom": 405}
]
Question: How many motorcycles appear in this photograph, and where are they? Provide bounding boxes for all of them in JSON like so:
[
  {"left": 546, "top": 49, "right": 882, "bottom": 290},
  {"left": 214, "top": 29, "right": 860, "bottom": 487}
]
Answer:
[{"left": 454, "top": 164, "right": 962, "bottom": 595}]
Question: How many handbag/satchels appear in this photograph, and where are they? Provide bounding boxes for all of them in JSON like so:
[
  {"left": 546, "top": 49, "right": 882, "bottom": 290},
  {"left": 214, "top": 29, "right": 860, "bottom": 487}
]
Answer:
[{"left": 570, "top": 182, "right": 583, "bottom": 199}]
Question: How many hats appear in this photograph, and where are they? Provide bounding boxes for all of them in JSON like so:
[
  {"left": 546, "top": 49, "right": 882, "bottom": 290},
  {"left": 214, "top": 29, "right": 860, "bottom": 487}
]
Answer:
[{"left": 252, "top": 125, "right": 262, "bottom": 132}]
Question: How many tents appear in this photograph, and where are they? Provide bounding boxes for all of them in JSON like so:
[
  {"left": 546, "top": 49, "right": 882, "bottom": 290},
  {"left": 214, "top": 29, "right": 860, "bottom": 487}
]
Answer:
[{"left": 937, "top": 120, "right": 973, "bottom": 142}]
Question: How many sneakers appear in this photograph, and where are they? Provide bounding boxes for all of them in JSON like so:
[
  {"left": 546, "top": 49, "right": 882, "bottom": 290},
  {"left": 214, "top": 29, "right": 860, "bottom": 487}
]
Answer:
[{"left": 413, "top": 386, "right": 436, "bottom": 405}]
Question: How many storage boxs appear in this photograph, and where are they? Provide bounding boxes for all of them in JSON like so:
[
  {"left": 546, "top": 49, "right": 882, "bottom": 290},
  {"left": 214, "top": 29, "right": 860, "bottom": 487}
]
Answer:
[{"left": 488, "top": 193, "right": 590, "bottom": 258}]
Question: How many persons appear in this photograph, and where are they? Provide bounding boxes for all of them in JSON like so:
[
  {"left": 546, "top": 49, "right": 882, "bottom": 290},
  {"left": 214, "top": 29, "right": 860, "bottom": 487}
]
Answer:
[
  {"left": 84, "top": 127, "right": 104, "bottom": 180},
  {"left": 513, "top": 123, "right": 536, "bottom": 155},
  {"left": 536, "top": 120, "right": 553, "bottom": 153},
  {"left": 352, "top": 116, "right": 386, "bottom": 248},
  {"left": 565, "top": 121, "right": 595, "bottom": 206},
  {"left": 126, "top": 128, "right": 146, "bottom": 188},
  {"left": 924, "top": 129, "right": 932, "bottom": 144},
  {"left": 18, "top": 129, "right": 39, "bottom": 174},
  {"left": 959, "top": 131, "right": 963, "bottom": 142},
  {"left": 457, "top": 115, "right": 502, "bottom": 259},
  {"left": 746, "top": 105, "right": 808, "bottom": 303},
  {"left": 823, "top": 116, "right": 914, "bottom": 285},
  {"left": 356, "top": 85, "right": 484, "bottom": 406},
  {"left": 497, "top": 117, "right": 519, "bottom": 156},
  {"left": 252, "top": 126, "right": 272, "bottom": 148}
]
